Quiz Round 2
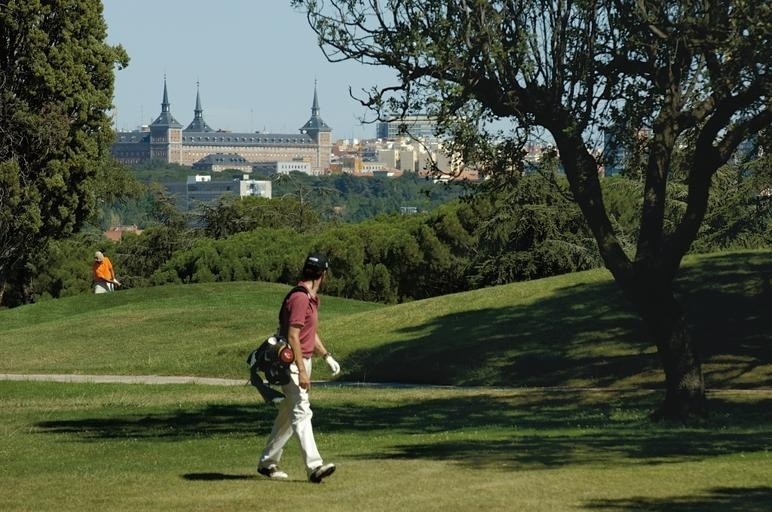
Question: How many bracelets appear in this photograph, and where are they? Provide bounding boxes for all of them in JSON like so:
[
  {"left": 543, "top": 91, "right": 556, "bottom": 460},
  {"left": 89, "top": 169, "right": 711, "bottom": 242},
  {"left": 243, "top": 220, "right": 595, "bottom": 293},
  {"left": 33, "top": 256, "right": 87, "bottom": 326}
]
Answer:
[
  {"left": 113, "top": 278, "right": 118, "bottom": 284},
  {"left": 323, "top": 352, "right": 331, "bottom": 359}
]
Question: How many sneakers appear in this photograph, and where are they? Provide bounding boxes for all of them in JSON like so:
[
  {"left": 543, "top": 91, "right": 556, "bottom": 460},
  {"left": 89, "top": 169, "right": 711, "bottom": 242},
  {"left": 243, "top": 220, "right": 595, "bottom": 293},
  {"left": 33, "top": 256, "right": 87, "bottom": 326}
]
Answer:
[
  {"left": 257, "top": 464, "right": 288, "bottom": 479},
  {"left": 311, "top": 463, "right": 335, "bottom": 483}
]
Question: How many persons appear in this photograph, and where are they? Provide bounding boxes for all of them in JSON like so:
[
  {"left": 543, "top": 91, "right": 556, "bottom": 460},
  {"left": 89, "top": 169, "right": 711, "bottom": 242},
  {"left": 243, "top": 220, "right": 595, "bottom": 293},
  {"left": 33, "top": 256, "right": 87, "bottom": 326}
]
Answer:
[
  {"left": 258, "top": 252, "right": 341, "bottom": 483},
  {"left": 94, "top": 250, "right": 121, "bottom": 293}
]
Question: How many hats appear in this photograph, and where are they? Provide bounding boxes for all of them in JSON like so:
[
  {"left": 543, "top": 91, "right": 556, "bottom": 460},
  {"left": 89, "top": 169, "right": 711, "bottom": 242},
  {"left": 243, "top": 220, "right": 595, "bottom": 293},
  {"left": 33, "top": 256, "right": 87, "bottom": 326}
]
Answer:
[
  {"left": 94, "top": 252, "right": 103, "bottom": 262},
  {"left": 306, "top": 251, "right": 329, "bottom": 268}
]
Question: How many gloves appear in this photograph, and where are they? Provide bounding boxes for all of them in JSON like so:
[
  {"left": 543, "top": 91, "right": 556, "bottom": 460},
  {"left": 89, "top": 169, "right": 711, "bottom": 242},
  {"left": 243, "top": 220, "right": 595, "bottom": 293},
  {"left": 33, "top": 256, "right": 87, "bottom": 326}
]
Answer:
[{"left": 325, "top": 356, "right": 341, "bottom": 376}]
